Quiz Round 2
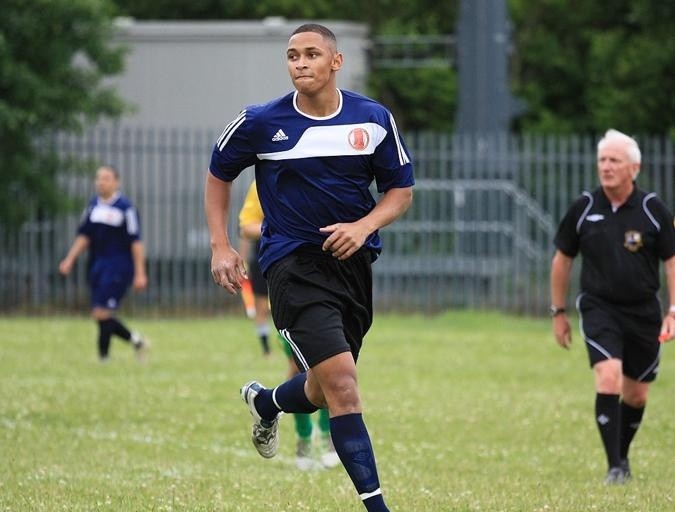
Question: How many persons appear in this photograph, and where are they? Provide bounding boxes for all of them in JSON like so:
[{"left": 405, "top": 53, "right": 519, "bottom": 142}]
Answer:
[
  {"left": 547, "top": 127, "right": 675, "bottom": 489},
  {"left": 277, "top": 334, "right": 341, "bottom": 474},
  {"left": 235, "top": 178, "right": 277, "bottom": 364},
  {"left": 205, "top": 24, "right": 416, "bottom": 511},
  {"left": 58, "top": 162, "right": 151, "bottom": 362}
]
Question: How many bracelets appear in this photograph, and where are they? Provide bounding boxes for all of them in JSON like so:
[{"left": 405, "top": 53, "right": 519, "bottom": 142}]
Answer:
[{"left": 668, "top": 305, "right": 675, "bottom": 312}]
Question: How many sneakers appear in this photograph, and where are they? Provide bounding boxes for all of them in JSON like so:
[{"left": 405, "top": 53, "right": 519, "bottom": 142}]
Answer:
[
  {"left": 602, "top": 466, "right": 624, "bottom": 486},
  {"left": 620, "top": 458, "right": 631, "bottom": 480},
  {"left": 239, "top": 380, "right": 283, "bottom": 459},
  {"left": 321, "top": 433, "right": 342, "bottom": 469},
  {"left": 295, "top": 423, "right": 315, "bottom": 472}
]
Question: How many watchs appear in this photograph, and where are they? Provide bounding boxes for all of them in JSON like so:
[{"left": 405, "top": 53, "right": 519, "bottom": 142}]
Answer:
[{"left": 549, "top": 305, "right": 566, "bottom": 316}]
